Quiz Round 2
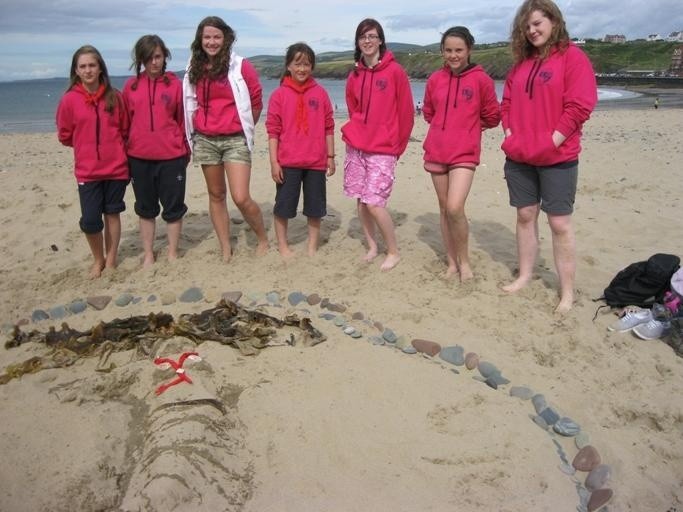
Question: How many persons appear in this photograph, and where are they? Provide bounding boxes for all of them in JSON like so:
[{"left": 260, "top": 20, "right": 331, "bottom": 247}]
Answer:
[
  {"left": 340, "top": 18, "right": 416, "bottom": 272},
  {"left": 54, "top": 44, "right": 132, "bottom": 279},
  {"left": 653, "top": 97, "right": 661, "bottom": 110},
  {"left": 332, "top": 101, "right": 338, "bottom": 114},
  {"left": 121, "top": 33, "right": 190, "bottom": 273},
  {"left": 499, "top": 0, "right": 598, "bottom": 318},
  {"left": 181, "top": 16, "right": 272, "bottom": 267},
  {"left": 420, "top": 26, "right": 502, "bottom": 284},
  {"left": 417, "top": 101, "right": 424, "bottom": 116},
  {"left": 264, "top": 40, "right": 335, "bottom": 261}
]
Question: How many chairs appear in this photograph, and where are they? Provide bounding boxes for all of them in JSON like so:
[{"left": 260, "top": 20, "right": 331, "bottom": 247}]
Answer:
[
  {"left": 608, "top": 309, "right": 653, "bottom": 333},
  {"left": 633, "top": 318, "right": 672, "bottom": 340}
]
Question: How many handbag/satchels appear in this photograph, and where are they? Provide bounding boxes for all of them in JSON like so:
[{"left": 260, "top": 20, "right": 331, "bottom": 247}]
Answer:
[{"left": 327, "top": 154, "right": 334, "bottom": 158}]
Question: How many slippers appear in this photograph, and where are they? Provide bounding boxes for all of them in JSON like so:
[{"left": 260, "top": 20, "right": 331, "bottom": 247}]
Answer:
[{"left": 604, "top": 253, "right": 680, "bottom": 307}]
